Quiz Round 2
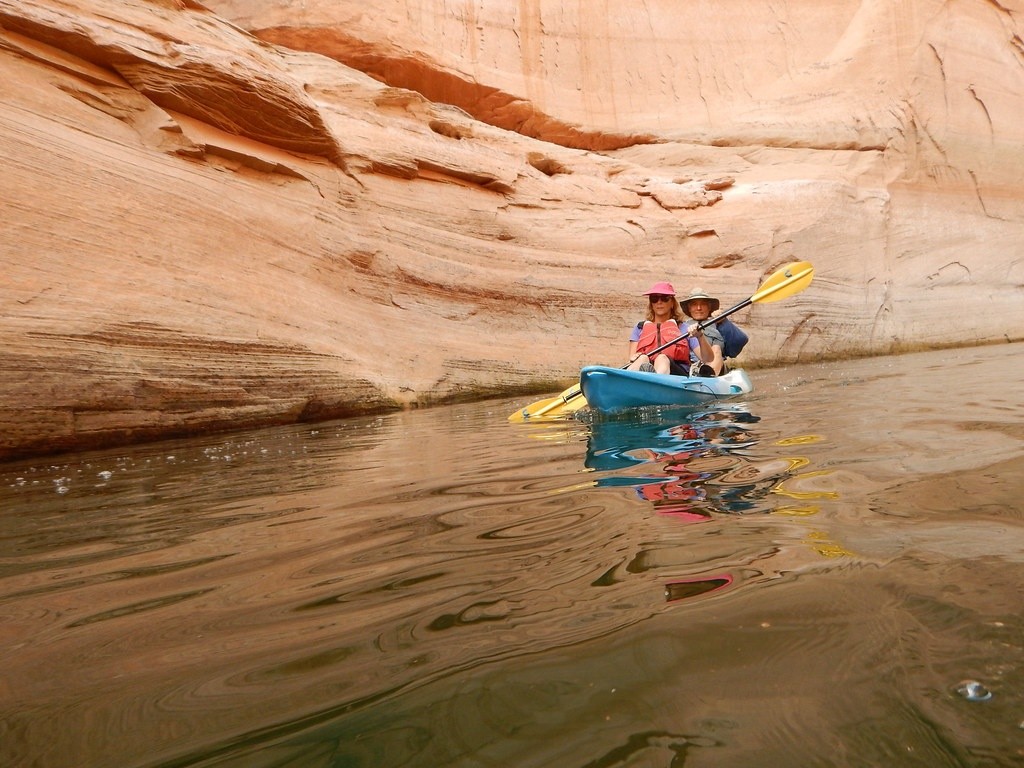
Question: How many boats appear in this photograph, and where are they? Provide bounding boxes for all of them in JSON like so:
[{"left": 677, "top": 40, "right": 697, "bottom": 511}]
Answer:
[{"left": 579, "top": 364, "right": 753, "bottom": 417}]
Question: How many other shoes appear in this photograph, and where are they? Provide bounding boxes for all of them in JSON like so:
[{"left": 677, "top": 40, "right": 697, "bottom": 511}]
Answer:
[
  {"left": 698, "top": 364, "right": 716, "bottom": 376},
  {"left": 639, "top": 363, "right": 656, "bottom": 372}
]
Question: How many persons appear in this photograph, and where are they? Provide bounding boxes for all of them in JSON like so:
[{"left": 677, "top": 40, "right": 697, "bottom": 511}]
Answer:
[
  {"left": 678, "top": 287, "right": 748, "bottom": 380},
  {"left": 629, "top": 282, "right": 714, "bottom": 378}
]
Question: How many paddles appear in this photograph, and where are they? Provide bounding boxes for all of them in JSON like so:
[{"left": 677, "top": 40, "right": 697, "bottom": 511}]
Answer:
[{"left": 506, "top": 260, "right": 813, "bottom": 421}]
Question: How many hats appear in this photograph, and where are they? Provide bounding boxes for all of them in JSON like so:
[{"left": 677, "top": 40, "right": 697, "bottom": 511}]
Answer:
[
  {"left": 642, "top": 282, "right": 675, "bottom": 296},
  {"left": 680, "top": 288, "right": 720, "bottom": 316}
]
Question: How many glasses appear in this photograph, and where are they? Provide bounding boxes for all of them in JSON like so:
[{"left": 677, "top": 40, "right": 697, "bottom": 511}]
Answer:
[{"left": 649, "top": 295, "right": 671, "bottom": 303}]
